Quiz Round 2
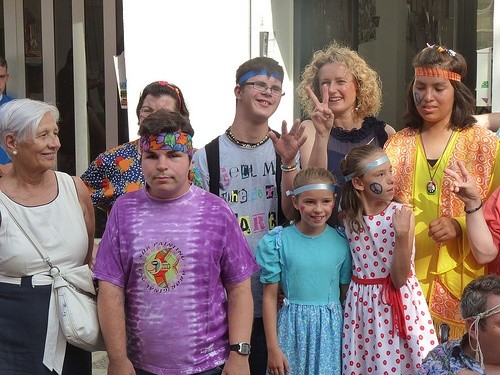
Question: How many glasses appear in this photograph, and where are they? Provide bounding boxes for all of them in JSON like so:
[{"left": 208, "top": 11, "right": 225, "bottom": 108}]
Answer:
[{"left": 244, "top": 81, "right": 287, "bottom": 97}]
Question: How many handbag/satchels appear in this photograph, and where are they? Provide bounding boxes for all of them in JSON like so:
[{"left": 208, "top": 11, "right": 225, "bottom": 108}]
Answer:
[{"left": 50, "top": 266, "right": 106, "bottom": 352}]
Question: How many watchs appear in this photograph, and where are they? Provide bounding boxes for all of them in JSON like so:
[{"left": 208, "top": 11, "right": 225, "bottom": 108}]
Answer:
[{"left": 228, "top": 342, "right": 252, "bottom": 355}]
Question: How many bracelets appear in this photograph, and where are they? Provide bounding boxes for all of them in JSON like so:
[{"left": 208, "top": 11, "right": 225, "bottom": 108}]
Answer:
[
  {"left": 280, "top": 162, "right": 298, "bottom": 172},
  {"left": 464, "top": 198, "right": 482, "bottom": 214}
]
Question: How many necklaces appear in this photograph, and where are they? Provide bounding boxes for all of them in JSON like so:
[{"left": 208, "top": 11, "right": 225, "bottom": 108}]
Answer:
[
  {"left": 419, "top": 120, "right": 456, "bottom": 196},
  {"left": 225, "top": 125, "right": 271, "bottom": 148}
]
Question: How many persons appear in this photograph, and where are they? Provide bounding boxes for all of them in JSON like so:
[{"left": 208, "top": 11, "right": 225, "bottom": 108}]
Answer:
[
  {"left": 335, "top": 145, "right": 440, "bottom": 375},
  {"left": 254, "top": 166, "right": 353, "bottom": 375},
  {"left": 0, "top": 39, "right": 500, "bottom": 375},
  {"left": 91, "top": 108, "right": 261, "bottom": 375},
  {"left": 0, "top": 97, "right": 96, "bottom": 375},
  {"left": 55, "top": 46, "right": 104, "bottom": 149}
]
